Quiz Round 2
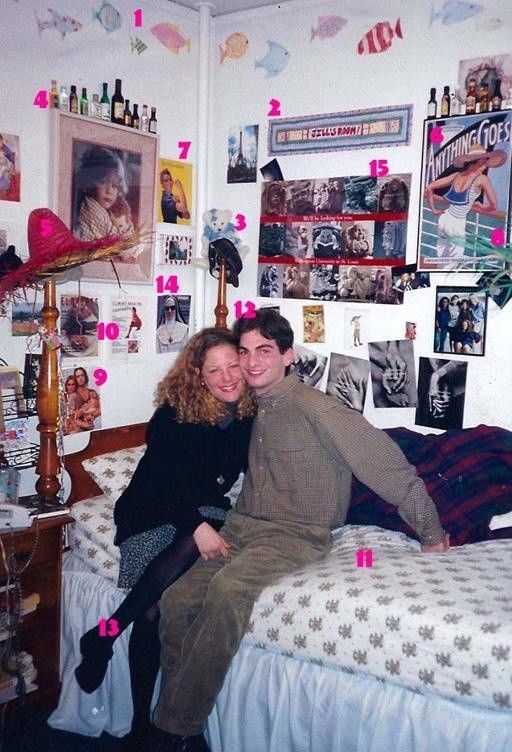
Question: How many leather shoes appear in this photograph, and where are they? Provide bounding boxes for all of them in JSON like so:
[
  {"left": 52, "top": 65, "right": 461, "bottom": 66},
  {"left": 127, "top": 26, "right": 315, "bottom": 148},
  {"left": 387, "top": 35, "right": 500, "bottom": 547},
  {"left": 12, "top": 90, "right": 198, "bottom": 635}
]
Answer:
[
  {"left": 74, "top": 624, "right": 114, "bottom": 694},
  {"left": 110, "top": 729, "right": 209, "bottom": 752}
]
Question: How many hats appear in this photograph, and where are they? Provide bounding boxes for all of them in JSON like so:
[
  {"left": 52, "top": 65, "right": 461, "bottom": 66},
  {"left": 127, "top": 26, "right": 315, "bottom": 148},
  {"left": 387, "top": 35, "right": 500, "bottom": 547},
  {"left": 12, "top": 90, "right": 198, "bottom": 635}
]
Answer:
[
  {"left": 0, "top": 206, "right": 119, "bottom": 286},
  {"left": 208, "top": 236, "right": 243, "bottom": 288},
  {"left": 451, "top": 143, "right": 508, "bottom": 170}
]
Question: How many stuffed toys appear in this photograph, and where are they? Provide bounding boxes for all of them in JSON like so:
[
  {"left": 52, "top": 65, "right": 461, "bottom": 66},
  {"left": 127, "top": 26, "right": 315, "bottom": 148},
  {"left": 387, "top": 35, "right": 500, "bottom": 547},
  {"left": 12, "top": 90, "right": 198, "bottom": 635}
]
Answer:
[{"left": 200, "top": 208, "right": 250, "bottom": 261}]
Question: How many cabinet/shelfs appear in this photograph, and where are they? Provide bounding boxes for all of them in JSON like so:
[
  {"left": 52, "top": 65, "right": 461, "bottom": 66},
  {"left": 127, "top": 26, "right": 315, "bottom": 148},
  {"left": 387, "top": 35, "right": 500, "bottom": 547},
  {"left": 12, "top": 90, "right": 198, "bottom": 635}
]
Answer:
[{"left": 0, "top": 357, "right": 40, "bottom": 472}]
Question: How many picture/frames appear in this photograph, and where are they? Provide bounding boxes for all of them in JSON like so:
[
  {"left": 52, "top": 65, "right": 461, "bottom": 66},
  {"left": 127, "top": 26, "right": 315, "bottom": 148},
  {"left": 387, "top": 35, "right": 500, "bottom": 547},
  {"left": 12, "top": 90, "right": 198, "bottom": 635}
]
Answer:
[
  {"left": 434, "top": 285, "right": 488, "bottom": 357},
  {"left": 412, "top": 106, "right": 512, "bottom": 274},
  {"left": 47, "top": 105, "right": 161, "bottom": 289},
  {"left": 160, "top": 234, "right": 194, "bottom": 266}
]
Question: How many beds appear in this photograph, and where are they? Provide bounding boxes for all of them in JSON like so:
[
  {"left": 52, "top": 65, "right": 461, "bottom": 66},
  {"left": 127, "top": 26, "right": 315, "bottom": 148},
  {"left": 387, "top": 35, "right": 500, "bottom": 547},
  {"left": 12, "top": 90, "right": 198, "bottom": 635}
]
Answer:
[{"left": 33, "top": 253, "right": 512, "bottom": 752}]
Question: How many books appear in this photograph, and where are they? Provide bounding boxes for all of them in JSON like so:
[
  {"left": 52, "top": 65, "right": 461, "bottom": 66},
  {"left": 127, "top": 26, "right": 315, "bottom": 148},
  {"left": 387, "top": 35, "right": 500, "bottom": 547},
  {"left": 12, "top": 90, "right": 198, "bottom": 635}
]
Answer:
[
  {"left": 18, "top": 494, "right": 70, "bottom": 520},
  {"left": 0, "top": 664, "right": 37, "bottom": 693}
]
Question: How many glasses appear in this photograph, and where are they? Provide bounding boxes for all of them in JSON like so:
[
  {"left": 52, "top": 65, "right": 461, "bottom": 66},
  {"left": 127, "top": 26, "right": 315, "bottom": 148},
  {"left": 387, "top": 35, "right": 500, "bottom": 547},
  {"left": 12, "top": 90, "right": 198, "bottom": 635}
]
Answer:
[{"left": 164, "top": 305, "right": 176, "bottom": 311}]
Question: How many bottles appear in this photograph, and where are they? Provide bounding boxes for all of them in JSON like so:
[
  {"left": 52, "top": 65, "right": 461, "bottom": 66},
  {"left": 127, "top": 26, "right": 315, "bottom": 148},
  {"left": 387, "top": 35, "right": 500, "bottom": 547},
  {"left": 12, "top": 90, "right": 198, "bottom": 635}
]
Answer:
[
  {"left": 49, "top": 75, "right": 159, "bottom": 135},
  {"left": 426, "top": 76, "right": 512, "bottom": 120}
]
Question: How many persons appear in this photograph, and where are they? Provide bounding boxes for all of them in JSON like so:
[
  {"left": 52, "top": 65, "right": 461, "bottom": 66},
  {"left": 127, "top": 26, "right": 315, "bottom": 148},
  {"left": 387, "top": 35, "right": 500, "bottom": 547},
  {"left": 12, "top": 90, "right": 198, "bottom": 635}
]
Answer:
[
  {"left": 137, "top": 309, "right": 450, "bottom": 752},
  {"left": 62, "top": 143, "right": 190, "bottom": 435},
  {"left": 74, "top": 328, "right": 257, "bottom": 752}
]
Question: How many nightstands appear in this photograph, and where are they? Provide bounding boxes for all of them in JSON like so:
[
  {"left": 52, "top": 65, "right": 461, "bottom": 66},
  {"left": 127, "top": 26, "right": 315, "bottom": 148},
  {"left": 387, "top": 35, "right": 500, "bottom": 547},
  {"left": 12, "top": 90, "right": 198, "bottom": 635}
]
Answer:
[{"left": 0, "top": 511, "right": 75, "bottom": 723}]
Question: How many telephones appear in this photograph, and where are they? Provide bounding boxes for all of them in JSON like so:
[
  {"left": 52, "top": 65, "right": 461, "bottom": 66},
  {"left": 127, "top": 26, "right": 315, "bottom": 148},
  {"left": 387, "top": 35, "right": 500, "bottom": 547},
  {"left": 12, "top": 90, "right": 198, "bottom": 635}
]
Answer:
[{"left": 0, "top": 504, "right": 34, "bottom": 529}]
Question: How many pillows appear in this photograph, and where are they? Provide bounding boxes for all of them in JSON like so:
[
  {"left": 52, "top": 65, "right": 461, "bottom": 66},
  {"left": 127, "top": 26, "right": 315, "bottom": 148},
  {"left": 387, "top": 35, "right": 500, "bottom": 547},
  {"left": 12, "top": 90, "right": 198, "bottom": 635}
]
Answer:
[{"left": 78, "top": 442, "right": 147, "bottom": 503}]
